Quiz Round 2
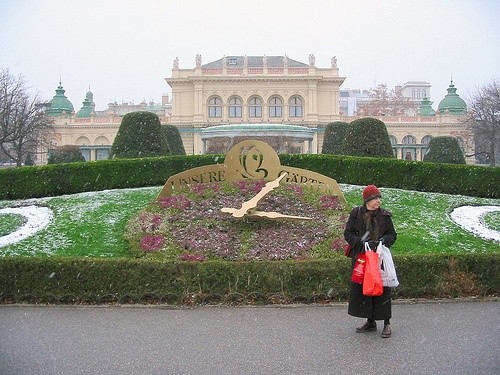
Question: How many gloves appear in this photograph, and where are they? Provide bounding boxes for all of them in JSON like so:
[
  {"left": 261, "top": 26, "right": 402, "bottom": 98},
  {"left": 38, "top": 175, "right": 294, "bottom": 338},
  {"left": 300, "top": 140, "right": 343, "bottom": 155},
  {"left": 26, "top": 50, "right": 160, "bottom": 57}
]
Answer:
[
  {"left": 377, "top": 238, "right": 384, "bottom": 245},
  {"left": 358, "top": 237, "right": 373, "bottom": 248}
]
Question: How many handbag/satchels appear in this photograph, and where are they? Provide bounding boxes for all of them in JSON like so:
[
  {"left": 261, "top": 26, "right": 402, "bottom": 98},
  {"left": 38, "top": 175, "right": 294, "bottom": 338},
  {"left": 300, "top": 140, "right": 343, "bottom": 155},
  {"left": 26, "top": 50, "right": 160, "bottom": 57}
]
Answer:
[
  {"left": 376, "top": 241, "right": 399, "bottom": 287},
  {"left": 343, "top": 207, "right": 361, "bottom": 258},
  {"left": 351, "top": 242, "right": 366, "bottom": 284},
  {"left": 363, "top": 242, "right": 383, "bottom": 296}
]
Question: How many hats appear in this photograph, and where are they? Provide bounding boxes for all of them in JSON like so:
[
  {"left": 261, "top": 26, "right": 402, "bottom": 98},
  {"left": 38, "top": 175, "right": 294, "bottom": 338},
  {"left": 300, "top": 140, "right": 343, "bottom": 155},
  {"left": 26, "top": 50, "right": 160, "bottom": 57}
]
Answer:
[{"left": 363, "top": 185, "right": 382, "bottom": 203}]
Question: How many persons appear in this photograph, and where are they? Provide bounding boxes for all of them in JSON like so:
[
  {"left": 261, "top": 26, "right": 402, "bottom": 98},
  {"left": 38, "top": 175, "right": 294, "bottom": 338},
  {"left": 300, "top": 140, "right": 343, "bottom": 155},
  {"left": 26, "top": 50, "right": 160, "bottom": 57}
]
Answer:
[{"left": 344, "top": 184, "right": 397, "bottom": 338}]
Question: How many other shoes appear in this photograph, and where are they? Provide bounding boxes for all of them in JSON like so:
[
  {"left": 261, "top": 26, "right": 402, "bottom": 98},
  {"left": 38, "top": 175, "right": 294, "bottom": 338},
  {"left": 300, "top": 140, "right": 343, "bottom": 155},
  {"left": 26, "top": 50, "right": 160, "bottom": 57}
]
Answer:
[
  {"left": 381, "top": 325, "right": 391, "bottom": 337},
  {"left": 356, "top": 321, "right": 377, "bottom": 333}
]
